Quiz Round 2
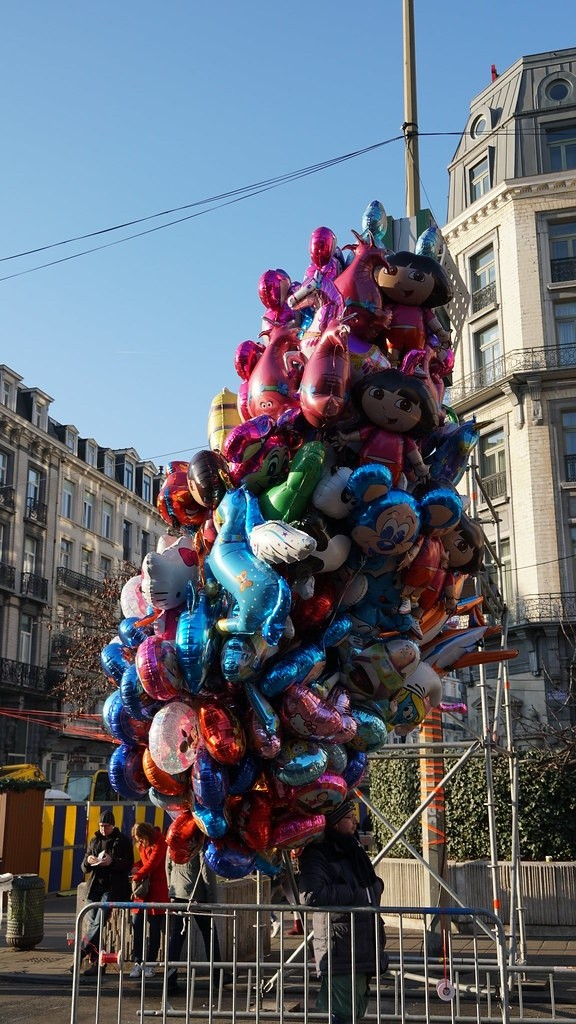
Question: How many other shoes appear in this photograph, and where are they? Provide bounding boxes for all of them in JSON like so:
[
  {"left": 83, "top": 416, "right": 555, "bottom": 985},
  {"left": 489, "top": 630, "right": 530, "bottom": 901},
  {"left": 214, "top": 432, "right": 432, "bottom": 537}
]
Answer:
[
  {"left": 84, "top": 966, "right": 106, "bottom": 976},
  {"left": 162, "top": 982, "right": 177, "bottom": 995},
  {"left": 70, "top": 960, "right": 82, "bottom": 973},
  {"left": 144, "top": 966, "right": 156, "bottom": 977},
  {"left": 215, "top": 973, "right": 233, "bottom": 990},
  {"left": 269, "top": 919, "right": 281, "bottom": 939},
  {"left": 130, "top": 963, "right": 142, "bottom": 977}
]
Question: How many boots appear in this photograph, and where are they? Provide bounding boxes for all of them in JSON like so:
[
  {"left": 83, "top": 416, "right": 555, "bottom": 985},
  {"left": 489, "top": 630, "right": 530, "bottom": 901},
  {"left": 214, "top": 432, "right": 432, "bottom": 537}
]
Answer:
[{"left": 287, "top": 919, "right": 303, "bottom": 935}]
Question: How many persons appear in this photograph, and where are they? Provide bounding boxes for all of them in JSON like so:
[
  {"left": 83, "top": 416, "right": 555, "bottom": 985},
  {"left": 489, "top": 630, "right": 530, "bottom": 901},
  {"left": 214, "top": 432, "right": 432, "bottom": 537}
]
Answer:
[
  {"left": 128, "top": 824, "right": 168, "bottom": 977},
  {"left": 69, "top": 809, "right": 134, "bottom": 975},
  {"left": 295, "top": 796, "right": 388, "bottom": 1024},
  {"left": 271, "top": 889, "right": 314, "bottom": 937},
  {"left": 163, "top": 841, "right": 238, "bottom": 987}
]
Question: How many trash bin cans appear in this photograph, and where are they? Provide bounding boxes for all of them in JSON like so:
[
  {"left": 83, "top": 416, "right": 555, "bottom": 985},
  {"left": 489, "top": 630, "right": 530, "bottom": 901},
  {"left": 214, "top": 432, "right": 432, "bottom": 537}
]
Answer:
[{"left": 5, "top": 872, "right": 46, "bottom": 946}]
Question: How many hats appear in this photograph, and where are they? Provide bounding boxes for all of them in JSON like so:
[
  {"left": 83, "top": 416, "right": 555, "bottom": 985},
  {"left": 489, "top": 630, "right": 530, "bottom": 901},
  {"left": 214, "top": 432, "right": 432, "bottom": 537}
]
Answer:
[
  {"left": 99, "top": 810, "right": 115, "bottom": 827},
  {"left": 324, "top": 794, "right": 356, "bottom": 827}
]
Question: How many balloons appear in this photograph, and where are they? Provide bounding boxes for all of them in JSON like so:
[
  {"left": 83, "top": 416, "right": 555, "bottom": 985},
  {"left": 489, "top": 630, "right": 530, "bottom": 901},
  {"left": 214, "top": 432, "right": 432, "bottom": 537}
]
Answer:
[{"left": 100, "top": 199, "right": 520, "bottom": 879}]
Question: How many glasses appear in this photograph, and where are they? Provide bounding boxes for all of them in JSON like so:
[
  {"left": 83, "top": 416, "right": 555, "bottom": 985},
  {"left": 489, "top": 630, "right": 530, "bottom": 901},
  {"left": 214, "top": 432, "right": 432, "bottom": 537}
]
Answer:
[
  {"left": 343, "top": 813, "right": 356, "bottom": 821},
  {"left": 100, "top": 824, "right": 112, "bottom": 829}
]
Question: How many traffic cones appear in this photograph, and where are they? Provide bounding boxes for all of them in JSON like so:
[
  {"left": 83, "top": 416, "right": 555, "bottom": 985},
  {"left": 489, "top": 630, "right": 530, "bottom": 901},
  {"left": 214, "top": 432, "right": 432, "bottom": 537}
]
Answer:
[{"left": 288, "top": 903, "right": 304, "bottom": 935}]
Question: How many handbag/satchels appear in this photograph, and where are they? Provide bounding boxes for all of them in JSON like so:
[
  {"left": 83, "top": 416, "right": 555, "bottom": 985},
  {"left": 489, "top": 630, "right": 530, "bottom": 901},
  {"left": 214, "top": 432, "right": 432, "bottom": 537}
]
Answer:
[{"left": 133, "top": 875, "right": 151, "bottom": 900}]
